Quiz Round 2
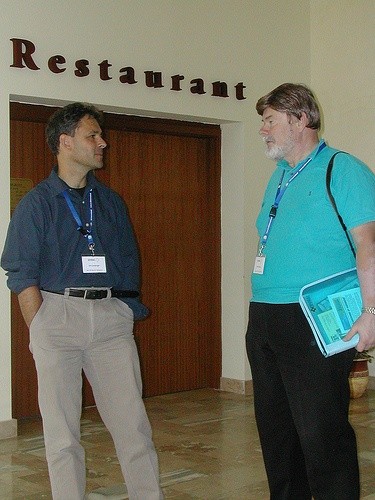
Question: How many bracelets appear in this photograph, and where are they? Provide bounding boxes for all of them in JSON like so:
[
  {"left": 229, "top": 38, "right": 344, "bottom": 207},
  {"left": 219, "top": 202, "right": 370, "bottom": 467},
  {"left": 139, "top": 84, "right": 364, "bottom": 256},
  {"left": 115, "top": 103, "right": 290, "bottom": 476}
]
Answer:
[{"left": 361, "top": 307, "right": 375, "bottom": 316}]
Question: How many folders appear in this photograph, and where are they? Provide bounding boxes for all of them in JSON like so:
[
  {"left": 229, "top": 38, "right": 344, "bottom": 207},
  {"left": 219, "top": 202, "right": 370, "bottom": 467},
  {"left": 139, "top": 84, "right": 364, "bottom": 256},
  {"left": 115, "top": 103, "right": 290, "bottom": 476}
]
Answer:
[{"left": 299, "top": 267, "right": 362, "bottom": 359}]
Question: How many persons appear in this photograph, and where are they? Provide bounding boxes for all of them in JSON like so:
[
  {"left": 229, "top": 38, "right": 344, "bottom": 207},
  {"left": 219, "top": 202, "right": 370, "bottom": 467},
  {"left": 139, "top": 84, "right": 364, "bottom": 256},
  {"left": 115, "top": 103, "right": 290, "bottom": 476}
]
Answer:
[
  {"left": 0, "top": 103, "right": 166, "bottom": 500},
  {"left": 245, "top": 83, "right": 375, "bottom": 500}
]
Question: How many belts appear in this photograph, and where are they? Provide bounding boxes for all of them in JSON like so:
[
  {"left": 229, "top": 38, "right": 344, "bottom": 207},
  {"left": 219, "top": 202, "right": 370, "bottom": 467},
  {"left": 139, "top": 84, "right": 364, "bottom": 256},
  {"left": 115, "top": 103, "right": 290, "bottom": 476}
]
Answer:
[{"left": 40, "top": 287, "right": 140, "bottom": 300}]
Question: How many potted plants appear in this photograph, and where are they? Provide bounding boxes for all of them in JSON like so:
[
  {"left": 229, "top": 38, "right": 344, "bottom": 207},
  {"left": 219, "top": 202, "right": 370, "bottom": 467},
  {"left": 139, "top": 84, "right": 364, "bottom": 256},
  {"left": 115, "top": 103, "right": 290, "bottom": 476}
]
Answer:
[{"left": 348, "top": 347, "right": 375, "bottom": 399}]
